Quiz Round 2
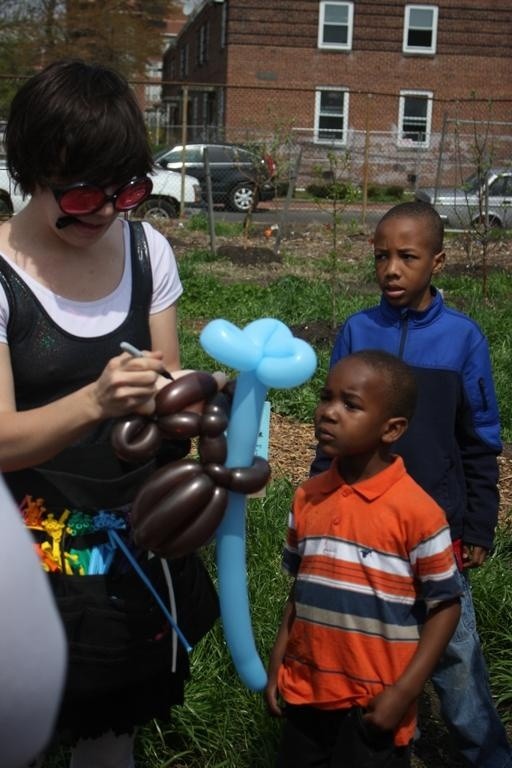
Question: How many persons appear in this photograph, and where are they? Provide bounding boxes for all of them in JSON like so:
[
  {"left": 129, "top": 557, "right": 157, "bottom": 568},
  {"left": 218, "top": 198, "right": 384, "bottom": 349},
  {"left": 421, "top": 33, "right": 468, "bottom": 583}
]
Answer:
[
  {"left": 264, "top": 352, "right": 462, "bottom": 768},
  {"left": 307, "top": 200, "right": 512, "bottom": 768},
  {"left": 0, "top": 59, "right": 220, "bottom": 767}
]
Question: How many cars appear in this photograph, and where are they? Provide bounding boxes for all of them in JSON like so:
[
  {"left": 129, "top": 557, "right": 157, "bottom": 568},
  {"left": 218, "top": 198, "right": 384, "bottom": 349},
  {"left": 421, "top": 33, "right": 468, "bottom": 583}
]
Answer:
[{"left": 415, "top": 165, "right": 512, "bottom": 234}]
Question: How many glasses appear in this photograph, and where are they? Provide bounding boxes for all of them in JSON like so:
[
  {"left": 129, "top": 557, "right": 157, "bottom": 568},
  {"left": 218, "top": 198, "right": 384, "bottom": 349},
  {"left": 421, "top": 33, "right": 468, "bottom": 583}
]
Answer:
[{"left": 44, "top": 170, "right": 154, "bottom": 217}]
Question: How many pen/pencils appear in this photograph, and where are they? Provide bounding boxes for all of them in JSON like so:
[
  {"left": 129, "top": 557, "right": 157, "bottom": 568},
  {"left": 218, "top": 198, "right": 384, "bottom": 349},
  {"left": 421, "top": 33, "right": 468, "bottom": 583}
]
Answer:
[{"left": 120, "top": 341, "right": 176, "bottom": 382}]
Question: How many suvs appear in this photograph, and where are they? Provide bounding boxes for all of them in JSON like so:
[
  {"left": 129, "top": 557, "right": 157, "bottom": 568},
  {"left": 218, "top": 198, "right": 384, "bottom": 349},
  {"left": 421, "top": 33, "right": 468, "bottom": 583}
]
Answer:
[
  {"left": 0, "top": 121, "right": 203, "bottom": 220},
  {"left": 151, "top": 139, "right": 278, "bottom": 213}
]
